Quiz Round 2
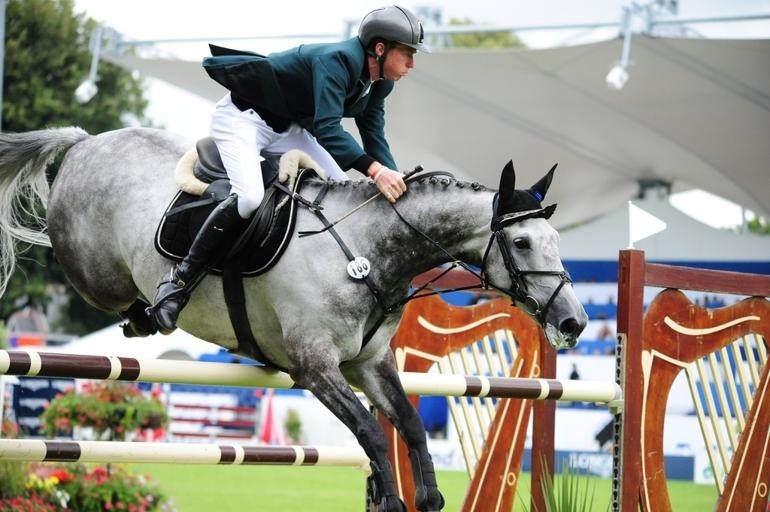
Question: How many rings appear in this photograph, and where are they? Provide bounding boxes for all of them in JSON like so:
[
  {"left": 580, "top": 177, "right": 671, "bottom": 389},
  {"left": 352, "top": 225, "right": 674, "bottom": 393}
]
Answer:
[{"left": 386, "top": 193, "right": 393, "bottom": 199}]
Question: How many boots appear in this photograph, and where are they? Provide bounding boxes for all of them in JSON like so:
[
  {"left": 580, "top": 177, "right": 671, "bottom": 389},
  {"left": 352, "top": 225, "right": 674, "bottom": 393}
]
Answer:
[{"left": 153, "top": 193, "right": 251, "bottom": 335}]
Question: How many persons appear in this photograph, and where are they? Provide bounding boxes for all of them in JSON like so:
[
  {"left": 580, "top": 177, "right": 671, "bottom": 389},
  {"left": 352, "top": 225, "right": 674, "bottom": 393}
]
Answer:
[{"left": 148, "top": 4, "right": 427, "bottom": 336}]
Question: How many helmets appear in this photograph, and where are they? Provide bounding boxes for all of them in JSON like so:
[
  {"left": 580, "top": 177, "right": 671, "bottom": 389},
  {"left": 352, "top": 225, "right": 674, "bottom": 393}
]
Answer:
[{"left": 359, "top": 6, "right": 432, "bottom": 56}]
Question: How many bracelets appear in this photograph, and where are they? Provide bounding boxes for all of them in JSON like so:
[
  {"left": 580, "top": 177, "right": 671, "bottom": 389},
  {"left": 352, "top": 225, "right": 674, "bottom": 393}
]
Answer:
[{"left": 371, "top": 164, "right": 387, "bottom": 183}]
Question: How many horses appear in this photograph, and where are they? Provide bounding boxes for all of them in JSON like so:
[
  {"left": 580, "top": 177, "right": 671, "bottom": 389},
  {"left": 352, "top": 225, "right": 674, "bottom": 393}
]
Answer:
[{"left": 0, "top": 125, "right": 590, "bottom": 512}]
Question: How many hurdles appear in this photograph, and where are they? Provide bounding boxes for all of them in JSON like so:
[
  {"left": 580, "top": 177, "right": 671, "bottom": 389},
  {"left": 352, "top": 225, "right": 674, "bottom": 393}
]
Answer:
[{"left": 0, "top": 248, "right": 770, "bottom": 512}]
